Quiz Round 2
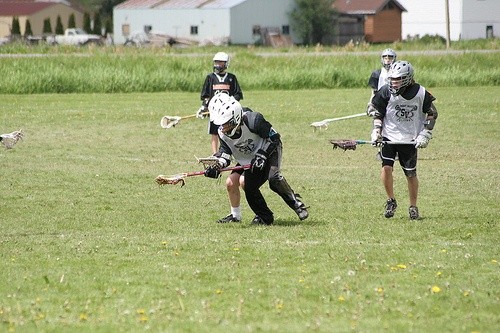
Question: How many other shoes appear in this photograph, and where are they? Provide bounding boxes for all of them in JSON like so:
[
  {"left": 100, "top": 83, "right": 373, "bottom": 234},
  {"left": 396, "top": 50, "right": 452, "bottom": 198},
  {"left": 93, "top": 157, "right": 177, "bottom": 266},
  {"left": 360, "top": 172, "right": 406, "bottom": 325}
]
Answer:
[
  {"left": 395, "top": 152, "right": 400, "bottom": 159},
  {"left": 376, "top": 153, "right": 382, "bottom": 160}
]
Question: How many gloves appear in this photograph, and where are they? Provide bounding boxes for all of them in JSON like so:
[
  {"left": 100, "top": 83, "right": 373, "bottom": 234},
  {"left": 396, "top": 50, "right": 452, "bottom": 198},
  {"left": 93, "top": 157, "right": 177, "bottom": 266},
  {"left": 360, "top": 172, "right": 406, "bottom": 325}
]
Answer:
[
  {"left": 367, "top": 103, "right": 378, "bottom": 116},
  {"left": 205, "top": 163, "right": 222, "bottom": 178},
  {"left": 414, "top": 130, "right": 433, "bottom": 148},
  {"left": 371, "top": 129, "right": 387, "bottom": 148},
  {"left": 250, "top": 149, "right": 271, "bottom": 180},
  {"left": 196, "top": 100, "right": 208, "bottom": 119}
]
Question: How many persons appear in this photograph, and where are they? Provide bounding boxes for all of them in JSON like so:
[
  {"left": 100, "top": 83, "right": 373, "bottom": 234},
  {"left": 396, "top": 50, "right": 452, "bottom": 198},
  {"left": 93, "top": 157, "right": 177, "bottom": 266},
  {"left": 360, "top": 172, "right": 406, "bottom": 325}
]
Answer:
[
  {"left": 367, "top": 48, "right": 397, "bottom": 117},
  {"left": 204, "top": 93, "right": 309, "bottom": 226},
  {"left": 196, "top": 52, "right": 244, "bottom": 155},
  {"left": 371, "top": 60, "right": 438, "bottom": 218}
]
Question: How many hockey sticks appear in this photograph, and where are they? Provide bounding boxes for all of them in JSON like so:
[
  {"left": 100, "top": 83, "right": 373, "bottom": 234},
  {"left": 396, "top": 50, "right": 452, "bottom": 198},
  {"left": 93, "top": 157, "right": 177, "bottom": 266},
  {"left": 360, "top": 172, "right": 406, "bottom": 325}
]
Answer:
[
  {"left": 153, "top": 163, "right": 255, "bottom": 188},
  {"left": 160, "top": 111, "right": 210, "bottom": 128},
  {"left": 0, "top": 130, "right": 23, "bottom": 148},
  {"left": 329, "top": 138, "right": 417, "bottom": 150},
  {"left": 309, "top": 112, "right": 376, "bottom": 134}
]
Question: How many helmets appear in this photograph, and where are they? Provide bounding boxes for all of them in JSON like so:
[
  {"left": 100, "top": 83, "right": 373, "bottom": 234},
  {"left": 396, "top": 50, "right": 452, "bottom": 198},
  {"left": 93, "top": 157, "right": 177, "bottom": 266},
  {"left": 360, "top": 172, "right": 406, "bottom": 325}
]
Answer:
[
  {"left": 387, "top": 60, "right": 414, "bottom": 96},
  {"left": 381, "top": 48, "right": 396, "bottom": 69},
  {"left": 208, "top": 92, "right": 230, "bottom": 122},
  {"left": 213, "top": 52, "right": 230, "bottom": 73},
  {"left": 213, "top": 95, "right": 242, "bottom": 137}
]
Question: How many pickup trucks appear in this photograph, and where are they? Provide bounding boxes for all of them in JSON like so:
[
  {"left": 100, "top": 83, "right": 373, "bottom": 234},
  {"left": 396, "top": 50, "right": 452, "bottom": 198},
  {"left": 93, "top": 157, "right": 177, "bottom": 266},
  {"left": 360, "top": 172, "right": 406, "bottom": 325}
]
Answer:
[{"left": 46, "top": 27, "right": 105, "bottom": 47}]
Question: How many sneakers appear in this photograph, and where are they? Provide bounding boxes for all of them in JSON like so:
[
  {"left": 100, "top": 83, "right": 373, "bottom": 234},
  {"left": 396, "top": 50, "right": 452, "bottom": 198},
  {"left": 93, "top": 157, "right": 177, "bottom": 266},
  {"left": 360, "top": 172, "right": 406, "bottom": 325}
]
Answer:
[
  {"left": 382, "top": 198, "right": 397, "bottom": 218},
  {"left": 295, "top": 201, "right": 308, "bottom": 220},
  {"left": 218, "top": 214, "right": 239, "bottom": 222},
  {"left": 409, "top": 205, "right": 418, "bottom": 219},
  {"left": 251, "top": 216, "right": 273, "bottom": 226}
]
